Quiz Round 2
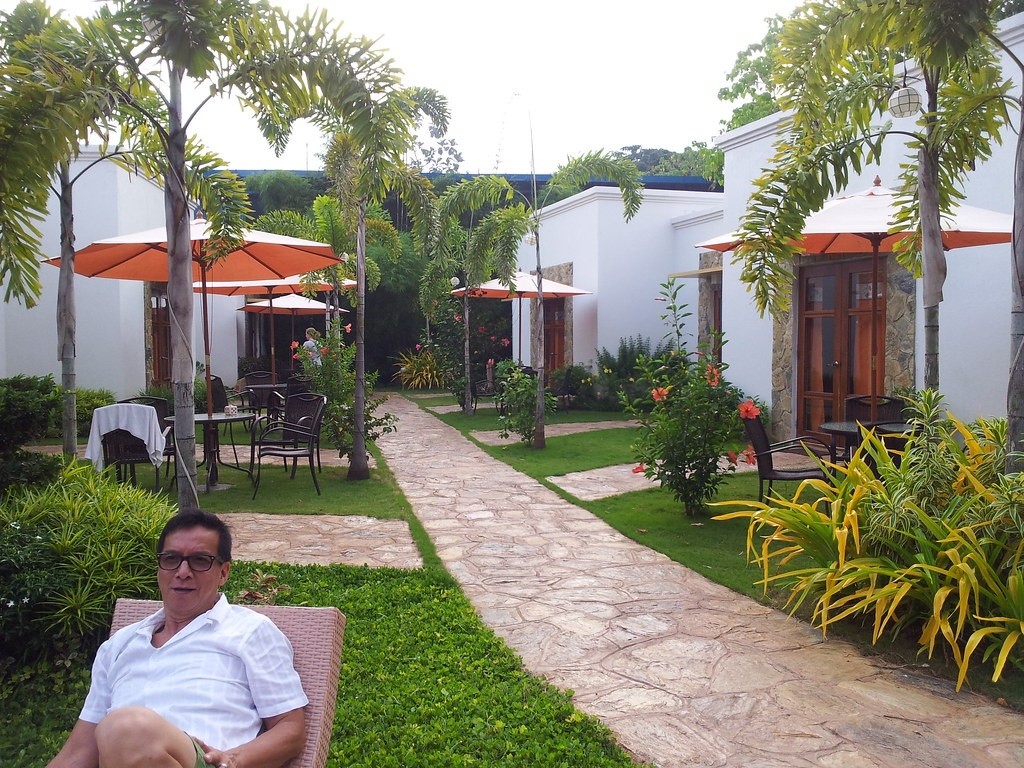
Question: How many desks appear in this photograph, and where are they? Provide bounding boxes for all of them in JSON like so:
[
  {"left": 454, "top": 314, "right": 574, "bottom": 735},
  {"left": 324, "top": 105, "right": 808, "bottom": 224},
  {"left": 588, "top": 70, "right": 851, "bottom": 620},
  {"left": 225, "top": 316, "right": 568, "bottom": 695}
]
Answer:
[
  {"left": 161, "top": 412, "right": 257, "bottom": 494},
  {"left": 819, "top": 419, "right": 882, "bottom": 440},
  {"left": 245, "top": 383, "right": 291, "bottom": 432}
]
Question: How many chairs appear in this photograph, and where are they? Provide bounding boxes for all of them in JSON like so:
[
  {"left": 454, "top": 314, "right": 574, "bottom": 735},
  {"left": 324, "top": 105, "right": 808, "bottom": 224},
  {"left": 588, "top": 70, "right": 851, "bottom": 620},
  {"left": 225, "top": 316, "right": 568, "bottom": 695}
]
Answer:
[
  {"left": 94, "top": 404, "right": 178, "bottom": 495},
  {"left": 252, "top": 395, "right": 328, "bottom": 499},
  {"left": 244, "top": 371, "right": 280, "bottom": 431},
  {"left": 204, "top": 375, "right": 264, "bottom": 436},
  {"left": 853, "top": 421, "right": 925, "bottom": 474},
  {"left": 469, "top": 364, "right": 500, "bottom": 415},
  {"left": 116, "top": 395, "right": 175, "bottom": 478},
  {"left": 266, "top": 375, "right": 319, "bottom": 433},
  {"left": 86, "top": 594, "right": 344, "bottom": 768},
  {"left": 834, "top": 394, "right": 905, "bottom": 463},
  {"left": 738, "top": 409, "right": 838, "bottom": 518},
  {"left": 544, "top": 365, "right": 574, "bottom": 415},
  {"left": 248, "top": 392, "right": 327, "bottom": 479}
]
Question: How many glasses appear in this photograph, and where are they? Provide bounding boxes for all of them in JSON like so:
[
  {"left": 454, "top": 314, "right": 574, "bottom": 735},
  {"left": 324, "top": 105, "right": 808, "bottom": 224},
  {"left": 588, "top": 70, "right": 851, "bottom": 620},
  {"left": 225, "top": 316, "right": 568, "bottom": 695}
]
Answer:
[{"left": 154, "top": 550, "right": 229, "bottom": 573}]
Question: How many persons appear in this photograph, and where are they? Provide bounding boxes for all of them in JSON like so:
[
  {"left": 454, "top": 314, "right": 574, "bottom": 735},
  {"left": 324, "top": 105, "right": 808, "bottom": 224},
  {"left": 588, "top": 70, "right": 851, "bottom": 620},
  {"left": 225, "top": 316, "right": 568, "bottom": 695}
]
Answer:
[
  {"left": 302, "top": 327, "right": 322, "bottom": 366},
  {"left": 42, "top": 511, "right": 309, "bottom": 768}
]
